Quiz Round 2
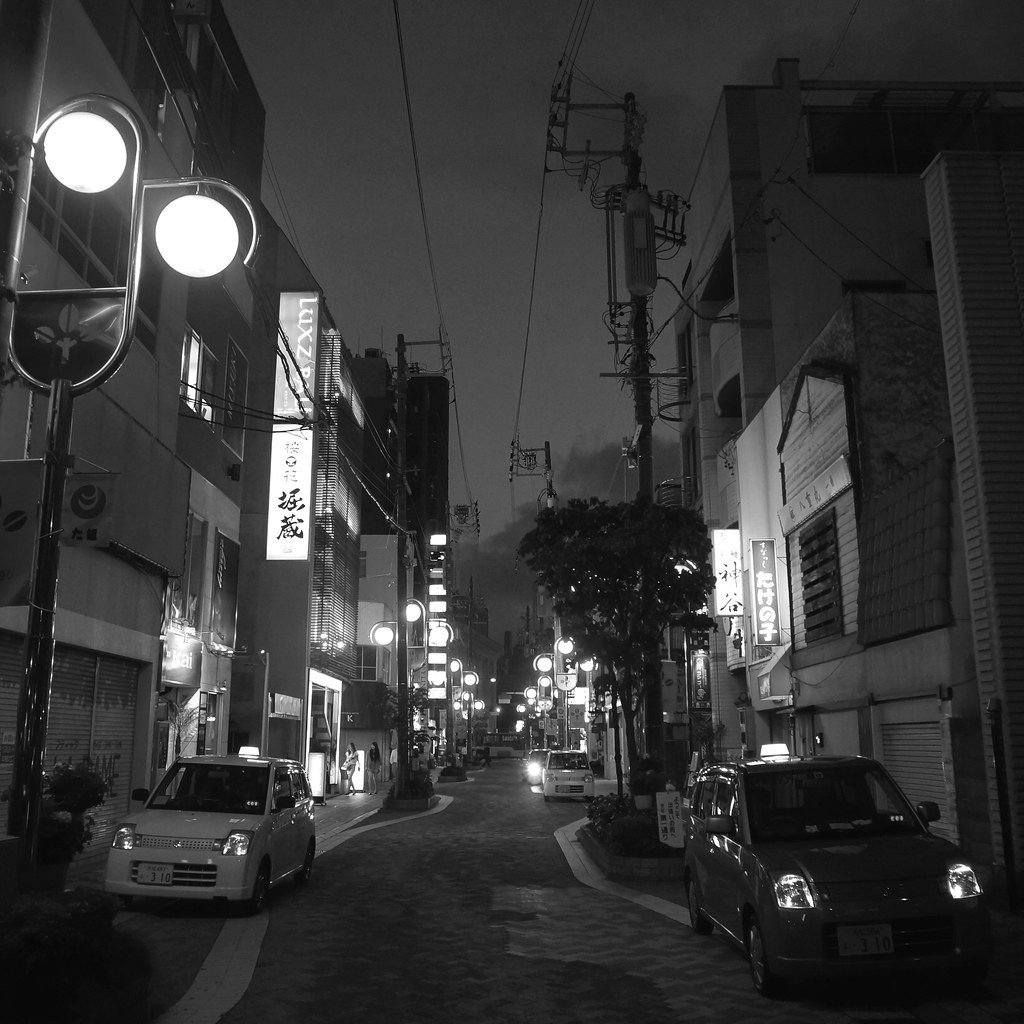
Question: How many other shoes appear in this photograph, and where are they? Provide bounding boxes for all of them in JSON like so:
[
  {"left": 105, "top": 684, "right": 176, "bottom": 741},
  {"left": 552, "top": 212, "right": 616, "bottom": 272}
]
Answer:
[
  {"left": 374, "top": 790, "right": 377, "bottom": 794},
  {"left": 368, "top": 791, "right": 373, "bottom": 795},
  {"left": 352, "top": 792, "right": 356, "bottom": 795},
  {"left": 345, "top": 790, "right": 351, "bottom": 796}
]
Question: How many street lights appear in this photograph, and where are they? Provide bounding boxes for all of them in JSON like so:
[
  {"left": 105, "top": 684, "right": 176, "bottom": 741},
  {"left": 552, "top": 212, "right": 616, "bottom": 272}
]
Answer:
[
  {"left": 4, "top": 90, "right": 265, "bottom": 901},
  {"left": 426, "top": 617, "right": 480, "bottom": 770},
  {"left": 365, "top": 594, "right": 429, "bottom": 777},
  {"left": 521, "top": 635, "right": 598, "bottom": 752}
]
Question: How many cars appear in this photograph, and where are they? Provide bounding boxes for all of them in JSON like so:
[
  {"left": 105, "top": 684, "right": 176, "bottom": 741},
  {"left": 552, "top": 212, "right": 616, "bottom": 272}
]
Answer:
[
  {"left": 103, "top": 745, "right": 316, "bottom": 916},
  {"left": 524, "top": 748, "right": 554, "bottom": 785},
  {"left": 541, "top": 750, "right": 597, "bottom": 804},
  {"left": 685, "top": 753, "right": 990, "bottom": 1002}
]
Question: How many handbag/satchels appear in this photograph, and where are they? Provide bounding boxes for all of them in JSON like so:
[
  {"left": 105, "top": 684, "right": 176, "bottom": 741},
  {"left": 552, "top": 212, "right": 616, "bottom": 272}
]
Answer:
[{"left": 341, "top": 762, "right": 349, "bottom": 771}]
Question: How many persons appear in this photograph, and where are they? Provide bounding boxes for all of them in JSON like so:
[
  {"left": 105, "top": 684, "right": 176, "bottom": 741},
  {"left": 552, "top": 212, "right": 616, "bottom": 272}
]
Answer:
[
  {"left": 390, "top": 749, "right": 398, "bottom": 780},
  {"left": 365, "top": 742, "right": 383, "bottom": 794},
  {"left": 345, "top": 743, "right": 360, "bottom": 795}
]
